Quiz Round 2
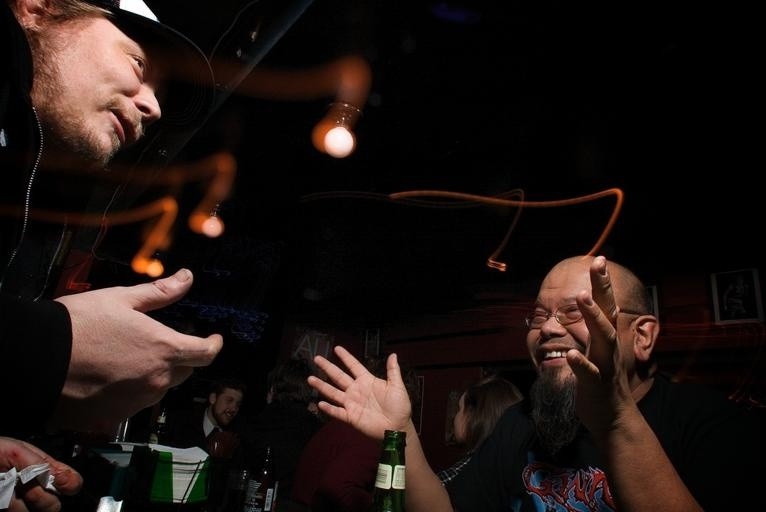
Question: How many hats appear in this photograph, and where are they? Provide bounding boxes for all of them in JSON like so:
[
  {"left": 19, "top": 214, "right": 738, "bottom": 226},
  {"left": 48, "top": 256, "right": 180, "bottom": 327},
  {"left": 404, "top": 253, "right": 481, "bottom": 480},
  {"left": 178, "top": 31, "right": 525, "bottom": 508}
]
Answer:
[{"left": 88, "top": 0, "right": 215, "bottom": 141}]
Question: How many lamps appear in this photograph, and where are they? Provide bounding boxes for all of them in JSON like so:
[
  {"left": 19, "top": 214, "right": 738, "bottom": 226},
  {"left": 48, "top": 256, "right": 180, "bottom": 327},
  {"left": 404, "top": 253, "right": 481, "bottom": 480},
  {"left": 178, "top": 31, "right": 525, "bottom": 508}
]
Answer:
[
  {"left": 325, "top": 102, "right": 364, "bottom": 156},
  {"left": 202, "top": 195, "right": 227, "bottom": 240}
]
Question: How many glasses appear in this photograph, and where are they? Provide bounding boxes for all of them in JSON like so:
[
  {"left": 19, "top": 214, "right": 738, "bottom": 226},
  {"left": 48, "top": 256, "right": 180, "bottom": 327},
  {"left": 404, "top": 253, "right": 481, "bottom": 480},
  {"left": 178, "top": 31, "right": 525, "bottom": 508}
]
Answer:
[{"left": 524, "top": 304, "right": 643, "bottom": 329}]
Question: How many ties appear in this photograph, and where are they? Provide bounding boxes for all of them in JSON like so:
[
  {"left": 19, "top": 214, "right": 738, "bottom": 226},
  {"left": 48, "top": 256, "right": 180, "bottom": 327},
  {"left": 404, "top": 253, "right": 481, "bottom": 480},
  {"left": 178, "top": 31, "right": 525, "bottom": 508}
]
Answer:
[{"left": 205, "top": 427, "right": 220, "bottom": 442}]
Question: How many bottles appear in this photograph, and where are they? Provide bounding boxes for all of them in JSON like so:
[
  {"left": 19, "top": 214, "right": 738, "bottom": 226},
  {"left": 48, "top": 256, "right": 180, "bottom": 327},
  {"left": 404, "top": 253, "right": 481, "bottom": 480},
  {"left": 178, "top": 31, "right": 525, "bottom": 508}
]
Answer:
[
  {"left": 367, "top": 429, "right": 408, "bottom": 512},
  {"left": 243, "top": 445, "right": 280, "bottom": 512},
  {"left": 149, "top": 405, "right": 169, "bottom": 444}
]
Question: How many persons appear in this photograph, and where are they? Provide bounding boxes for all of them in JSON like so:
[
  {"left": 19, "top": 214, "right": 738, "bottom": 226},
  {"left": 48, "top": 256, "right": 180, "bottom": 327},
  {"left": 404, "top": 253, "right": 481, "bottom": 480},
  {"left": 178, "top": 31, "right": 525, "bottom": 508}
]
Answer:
[
  {"left": 0, "top": 1, "right": 227, "bottom": 512},
  {"left": 452, "top": 364, "right": 526, "bottom": 448},
  {"left": 164, "top": 375, "right": 244, "bottom": 463},
  {"left": 236, "top": 372, "right": 323, "bottom": 471},
  {"left": 305, "top": 252, "right": 766, "bottom": 512}
]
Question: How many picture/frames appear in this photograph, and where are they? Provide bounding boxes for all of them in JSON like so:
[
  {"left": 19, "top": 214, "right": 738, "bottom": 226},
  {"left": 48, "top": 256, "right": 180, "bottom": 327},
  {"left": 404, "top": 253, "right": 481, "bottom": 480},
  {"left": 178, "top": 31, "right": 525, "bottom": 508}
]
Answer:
[{"left": 705, "top": 264, "right": 766, "bottom": 326}]
